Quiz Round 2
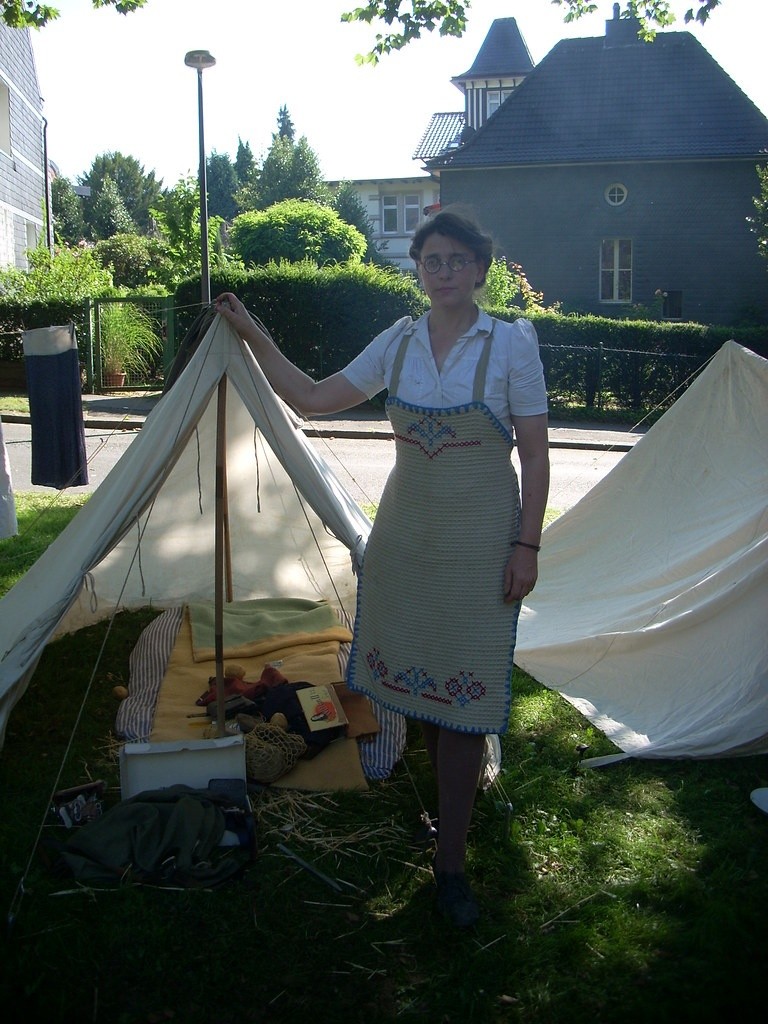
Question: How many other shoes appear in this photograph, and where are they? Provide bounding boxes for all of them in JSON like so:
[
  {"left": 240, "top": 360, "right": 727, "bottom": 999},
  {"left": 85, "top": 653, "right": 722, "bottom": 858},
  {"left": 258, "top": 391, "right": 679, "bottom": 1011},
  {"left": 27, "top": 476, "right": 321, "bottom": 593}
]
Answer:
[{"left": 434, "top": 850, "right": 480, "bottom": 928}]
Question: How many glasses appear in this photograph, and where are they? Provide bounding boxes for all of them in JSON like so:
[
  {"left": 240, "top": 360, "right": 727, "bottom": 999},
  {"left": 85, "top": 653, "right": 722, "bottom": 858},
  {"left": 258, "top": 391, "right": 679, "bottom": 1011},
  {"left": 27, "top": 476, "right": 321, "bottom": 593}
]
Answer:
[{"left": 418, "top": 252, "right": 476, "bottom": 275}]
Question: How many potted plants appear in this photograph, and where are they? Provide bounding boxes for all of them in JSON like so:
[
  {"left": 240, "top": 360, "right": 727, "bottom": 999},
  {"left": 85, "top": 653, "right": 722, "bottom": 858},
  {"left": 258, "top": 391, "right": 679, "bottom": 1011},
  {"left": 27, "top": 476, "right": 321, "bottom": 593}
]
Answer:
[{"left": 98, "top": 289, "right": 165, "bottom": 386}]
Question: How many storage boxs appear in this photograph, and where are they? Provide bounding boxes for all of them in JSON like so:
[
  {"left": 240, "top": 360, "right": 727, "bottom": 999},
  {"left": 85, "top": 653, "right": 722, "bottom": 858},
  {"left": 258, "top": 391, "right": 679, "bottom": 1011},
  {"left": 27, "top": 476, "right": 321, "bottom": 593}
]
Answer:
[
  {"left": 117, "top": 733, "right": 259, "bottom": 871},
  {"left": 295, "top": 681, "right": 381, "bottom": 748}
]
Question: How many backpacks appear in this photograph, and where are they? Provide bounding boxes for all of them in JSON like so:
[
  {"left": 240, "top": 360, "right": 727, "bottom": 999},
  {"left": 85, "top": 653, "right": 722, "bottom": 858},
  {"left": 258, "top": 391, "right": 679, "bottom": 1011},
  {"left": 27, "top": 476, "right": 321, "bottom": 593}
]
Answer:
[{"left": 60, "top": 785, "right": 255, "bottom": 892}]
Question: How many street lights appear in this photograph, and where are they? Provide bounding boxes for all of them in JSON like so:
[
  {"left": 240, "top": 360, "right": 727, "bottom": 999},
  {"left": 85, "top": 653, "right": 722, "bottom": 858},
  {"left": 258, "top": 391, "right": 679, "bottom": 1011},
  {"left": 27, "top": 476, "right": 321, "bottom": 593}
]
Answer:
[{"left": 183, "top": 48, "right": 217, "bottom": 302}]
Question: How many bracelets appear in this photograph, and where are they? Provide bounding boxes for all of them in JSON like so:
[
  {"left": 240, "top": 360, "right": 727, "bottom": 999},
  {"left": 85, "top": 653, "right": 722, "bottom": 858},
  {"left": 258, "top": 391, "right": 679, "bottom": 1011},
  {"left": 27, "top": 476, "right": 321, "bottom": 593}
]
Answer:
[{"left": 510, "top": 540, "right": 543, "bottom": 552}]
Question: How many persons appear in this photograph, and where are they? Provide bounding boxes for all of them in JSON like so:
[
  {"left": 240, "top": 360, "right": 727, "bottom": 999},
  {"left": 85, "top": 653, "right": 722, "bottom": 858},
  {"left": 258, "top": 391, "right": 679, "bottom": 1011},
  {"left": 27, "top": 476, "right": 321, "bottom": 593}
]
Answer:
[{"left": 213, "top": 211, "right": 549, "bottom": 900}]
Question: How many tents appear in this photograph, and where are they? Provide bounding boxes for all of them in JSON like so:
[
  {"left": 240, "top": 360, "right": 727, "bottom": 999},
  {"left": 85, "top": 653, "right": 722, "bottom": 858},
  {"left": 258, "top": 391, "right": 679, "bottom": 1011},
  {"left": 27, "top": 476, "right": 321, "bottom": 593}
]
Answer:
[
  {"left": 0, "top": 296, "right": 375, "bottom": 720},
  {"left": 512, "top": 338, "right": 768, "bottom": 758}
]
